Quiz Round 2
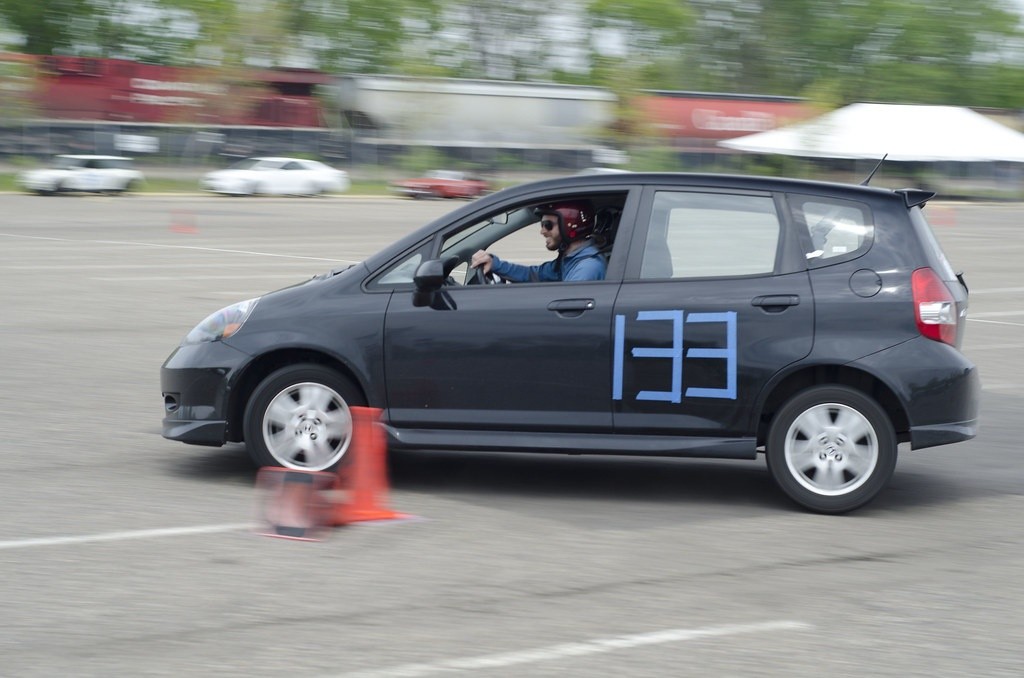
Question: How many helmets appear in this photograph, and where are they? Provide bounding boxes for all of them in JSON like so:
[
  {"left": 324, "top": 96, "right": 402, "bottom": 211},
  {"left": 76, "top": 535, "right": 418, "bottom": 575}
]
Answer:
[{"left": 534, "top": 201, "right": 595, "bottom": 244}]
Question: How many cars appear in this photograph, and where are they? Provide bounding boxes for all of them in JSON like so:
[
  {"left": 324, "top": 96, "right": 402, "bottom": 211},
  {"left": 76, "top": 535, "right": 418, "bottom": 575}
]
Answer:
[
  {"left": 158, "top": 154, "right": 981, "bottom": 514},
  {"left": 25, "top": 154, "right": 142, "bottom": 195},
  {"left": 199, "top": 156, "right": 349, "bottom": 197},
  {"left": 387, "top": 169, "right": 490, "bottom": 201}
]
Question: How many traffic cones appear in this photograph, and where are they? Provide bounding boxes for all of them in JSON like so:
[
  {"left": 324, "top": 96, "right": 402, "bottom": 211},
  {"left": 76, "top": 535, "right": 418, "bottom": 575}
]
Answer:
[{"left": 322, "top": 407, "right": 407, "bottom": 523}]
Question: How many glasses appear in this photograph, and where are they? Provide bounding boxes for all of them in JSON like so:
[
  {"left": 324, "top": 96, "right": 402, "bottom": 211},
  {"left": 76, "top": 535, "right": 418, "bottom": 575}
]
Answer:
[{"left": 541, "top": 220, "right": 558, "bottom": 231}]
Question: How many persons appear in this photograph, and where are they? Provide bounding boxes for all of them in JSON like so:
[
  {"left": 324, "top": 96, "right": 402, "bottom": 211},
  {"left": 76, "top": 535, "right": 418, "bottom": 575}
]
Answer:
[{"left": 470, "top": 200, "right": 607, "bottom": 282}]
